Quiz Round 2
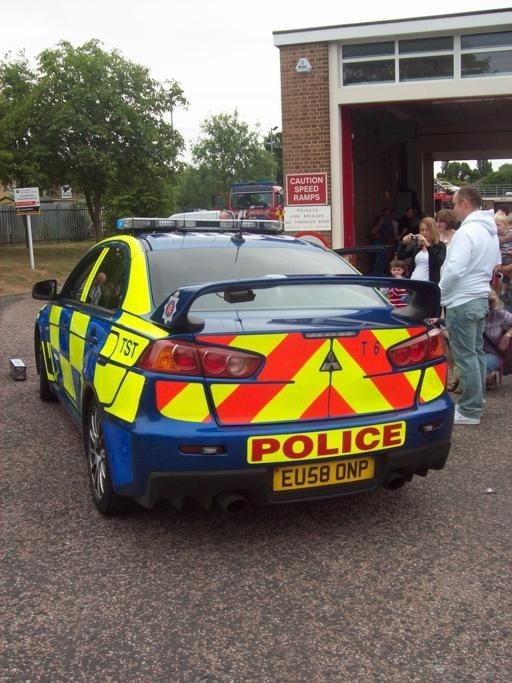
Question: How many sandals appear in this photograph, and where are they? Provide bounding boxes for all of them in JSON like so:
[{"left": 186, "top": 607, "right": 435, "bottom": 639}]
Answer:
[{"left": 447, "top": 377, "right": 458, "bottom": 393}]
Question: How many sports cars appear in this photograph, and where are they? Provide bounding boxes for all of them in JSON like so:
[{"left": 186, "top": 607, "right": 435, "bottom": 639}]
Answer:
[{"left": 31, "top": 217, "right": 456, "bottom": 520}]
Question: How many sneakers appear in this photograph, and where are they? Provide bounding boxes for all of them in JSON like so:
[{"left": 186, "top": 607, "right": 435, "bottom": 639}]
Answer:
[{"left": 453, "top": 404, "right": 481, "bottom": 426}]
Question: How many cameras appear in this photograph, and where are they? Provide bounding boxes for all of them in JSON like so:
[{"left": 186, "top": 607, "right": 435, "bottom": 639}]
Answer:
[{"left": 411, "top": 235, "right": 418, "bottom": 242}]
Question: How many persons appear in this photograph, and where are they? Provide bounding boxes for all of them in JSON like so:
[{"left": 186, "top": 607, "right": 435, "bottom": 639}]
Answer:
[
  {"left": 370, "top": 187, "right": 511, "bottom": 425},
  {"left": 77, "top": 271, "right": 108, "bottom": 305}
]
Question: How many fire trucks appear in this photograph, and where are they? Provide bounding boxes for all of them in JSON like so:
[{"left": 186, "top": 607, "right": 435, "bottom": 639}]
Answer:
[{"left": 227, "top": 182, "right": 285, "bottom": 220}]
